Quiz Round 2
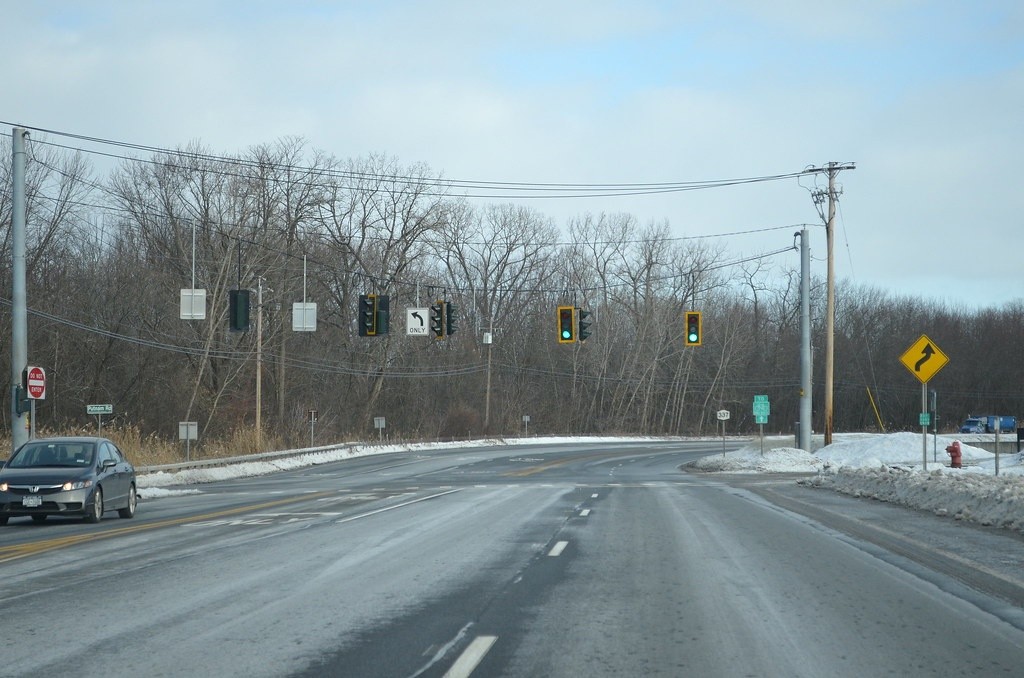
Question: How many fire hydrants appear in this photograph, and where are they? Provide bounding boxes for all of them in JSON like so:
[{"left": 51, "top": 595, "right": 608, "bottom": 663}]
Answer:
[{"left": 947, "top": 442, "right": 962, "bottom": 468}]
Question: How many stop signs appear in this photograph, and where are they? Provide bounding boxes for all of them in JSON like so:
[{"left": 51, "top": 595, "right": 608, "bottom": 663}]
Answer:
[{"left": 26, "top": 367, "right": 46, "bottom": 399}]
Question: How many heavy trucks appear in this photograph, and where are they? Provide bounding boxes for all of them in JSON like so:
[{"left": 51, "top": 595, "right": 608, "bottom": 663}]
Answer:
[{"left": 962, "top": 415, "right": 1016, "bottom": 436}]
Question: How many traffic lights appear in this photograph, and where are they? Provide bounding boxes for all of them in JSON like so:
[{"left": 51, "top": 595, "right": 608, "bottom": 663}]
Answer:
[
  {"left": 431, "top": 300, "right": 444, "bottom": 338},
  {"left": 579, "top": 310, "right": 591, "bottom": 340},
  {"left": 685, "top": 312, "right": 701, "bottom": 345},
  {"left": 557, "top": 306, "right": 576, "bottom": 343},
  {"left": 447, "top": 302, "right": 460, "bottom": 336},
  {"left": 364, "top": 294, "right": 376, "bottom": 335}
]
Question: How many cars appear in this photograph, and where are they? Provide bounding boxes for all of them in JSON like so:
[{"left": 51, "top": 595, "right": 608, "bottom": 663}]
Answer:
[{"left": 0, "top": 438, "right": 137, "bottom": 524}]
